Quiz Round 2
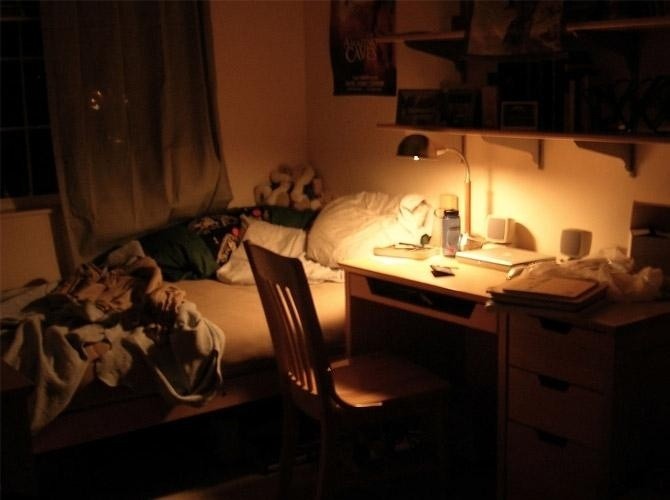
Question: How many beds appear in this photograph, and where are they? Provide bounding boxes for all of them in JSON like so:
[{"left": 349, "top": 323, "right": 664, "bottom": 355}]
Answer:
[{"left": 1, "top": 204, "right": 346, "bottom": 500}]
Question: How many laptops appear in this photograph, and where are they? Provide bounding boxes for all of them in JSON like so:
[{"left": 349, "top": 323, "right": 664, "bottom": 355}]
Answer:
[{"left": 454, "top": 246, "right": 556, "bottom": 271}]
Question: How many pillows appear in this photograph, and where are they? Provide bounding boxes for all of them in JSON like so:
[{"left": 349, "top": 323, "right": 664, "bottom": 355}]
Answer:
[
  {"left": 308, "top": 190, "right": 436, "bottom": 266},
  {"left": 217, "top": 222, "right": 307, "bottom": 282}
]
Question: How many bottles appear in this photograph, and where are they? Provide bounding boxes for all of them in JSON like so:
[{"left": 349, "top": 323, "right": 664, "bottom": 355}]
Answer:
[{"left": 434, "top": 207, "right": 459, "bottom": 256}]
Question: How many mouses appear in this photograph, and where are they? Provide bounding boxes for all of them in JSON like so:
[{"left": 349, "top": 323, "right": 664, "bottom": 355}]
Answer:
[{"left": 508, "top": 267, "right": 524, "bottom": 279}]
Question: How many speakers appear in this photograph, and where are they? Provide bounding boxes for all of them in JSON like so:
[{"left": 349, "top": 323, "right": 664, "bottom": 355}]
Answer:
[
  {"left": 482, "top": 215, "right": 515, "bottom": 249},
  {"left": 560, "top": 228, "right": 592, "bottom": 266}
]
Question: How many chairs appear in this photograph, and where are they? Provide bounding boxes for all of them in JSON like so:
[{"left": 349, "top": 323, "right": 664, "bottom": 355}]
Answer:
[{"left": 246, "top": 240, "right": 452, "bottom": 500}]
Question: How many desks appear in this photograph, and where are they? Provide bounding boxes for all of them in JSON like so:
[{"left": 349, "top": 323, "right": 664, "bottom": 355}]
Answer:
[{"left": 337, "top": 244, "right": 670, "bottom": 500}]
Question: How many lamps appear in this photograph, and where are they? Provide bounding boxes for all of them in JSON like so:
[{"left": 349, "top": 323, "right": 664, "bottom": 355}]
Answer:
[{"left": 396, "top": 134, "right": 487, "bottom": 250}]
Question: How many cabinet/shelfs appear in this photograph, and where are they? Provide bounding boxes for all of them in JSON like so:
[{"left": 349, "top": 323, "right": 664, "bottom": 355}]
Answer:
[{"left": 376, "top": 16, "right": 670, "bottom": 179}]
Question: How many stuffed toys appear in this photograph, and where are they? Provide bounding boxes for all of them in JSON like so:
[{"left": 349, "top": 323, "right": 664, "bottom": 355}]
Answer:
[{"left": 254, "top": 162, "right": 322, "bottom": 210}]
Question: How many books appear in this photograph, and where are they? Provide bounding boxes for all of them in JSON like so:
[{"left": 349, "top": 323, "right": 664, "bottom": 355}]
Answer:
[
  {"left": 373, "top": 242, "right": 439, "bottom": 260},
  {"left": 485, "top": 275, "right": 608, "bottom": 312}
]
